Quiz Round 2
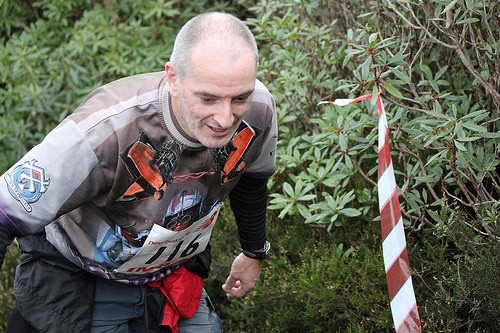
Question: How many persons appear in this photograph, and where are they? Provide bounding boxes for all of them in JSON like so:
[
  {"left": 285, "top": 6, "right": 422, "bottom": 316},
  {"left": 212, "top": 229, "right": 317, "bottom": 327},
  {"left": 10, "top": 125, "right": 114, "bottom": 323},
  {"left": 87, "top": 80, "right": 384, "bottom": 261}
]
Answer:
[{"left": 0, "top": 12, "right": 278, "bottom": 333}]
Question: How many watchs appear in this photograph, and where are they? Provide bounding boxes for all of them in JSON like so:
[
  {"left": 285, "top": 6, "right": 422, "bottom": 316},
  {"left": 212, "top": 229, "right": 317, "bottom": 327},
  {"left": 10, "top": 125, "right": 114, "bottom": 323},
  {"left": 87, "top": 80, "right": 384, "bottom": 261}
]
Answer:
[{"left": 242, "top": 241, "right": 271, "bottom": 259}]
